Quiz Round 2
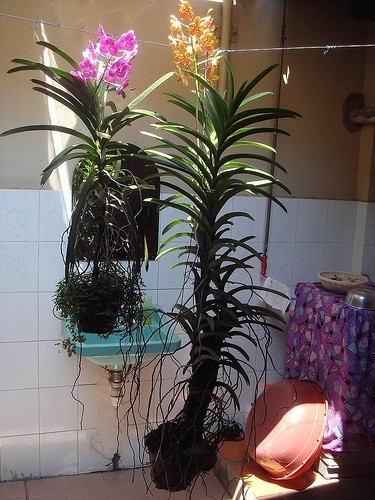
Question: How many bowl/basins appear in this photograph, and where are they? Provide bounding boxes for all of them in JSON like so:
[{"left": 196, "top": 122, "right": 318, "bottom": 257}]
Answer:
[
  {"left": 244, "top": 380, "right": 329, "bottom": 480},
  {"left": 343, "top": 288, "right": 375, "bottom": 310}
]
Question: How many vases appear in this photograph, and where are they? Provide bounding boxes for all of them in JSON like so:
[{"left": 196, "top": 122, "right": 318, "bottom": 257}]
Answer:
[{"left": 70, "top": 285, "right": 123, "bottom": 335}]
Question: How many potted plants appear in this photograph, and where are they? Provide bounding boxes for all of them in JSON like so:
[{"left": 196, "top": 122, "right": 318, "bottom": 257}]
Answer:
[{"left": 136, "top": 51, "right": 303, "bottom": 493}]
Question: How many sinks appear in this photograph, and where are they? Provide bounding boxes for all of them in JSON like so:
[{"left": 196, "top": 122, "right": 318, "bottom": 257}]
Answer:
[{"left": 62, "top": 307, "right": 183, "bottom": 371}]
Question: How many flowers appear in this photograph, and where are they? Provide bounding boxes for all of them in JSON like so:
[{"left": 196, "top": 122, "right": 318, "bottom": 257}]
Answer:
[{"left": 3, "top": 21, "right": 177, "bottom": 285}]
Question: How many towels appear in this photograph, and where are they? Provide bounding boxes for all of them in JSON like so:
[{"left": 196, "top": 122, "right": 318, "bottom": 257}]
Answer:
[{"left": 257, "top": 276, "right": 298, "bottom": 316}]
